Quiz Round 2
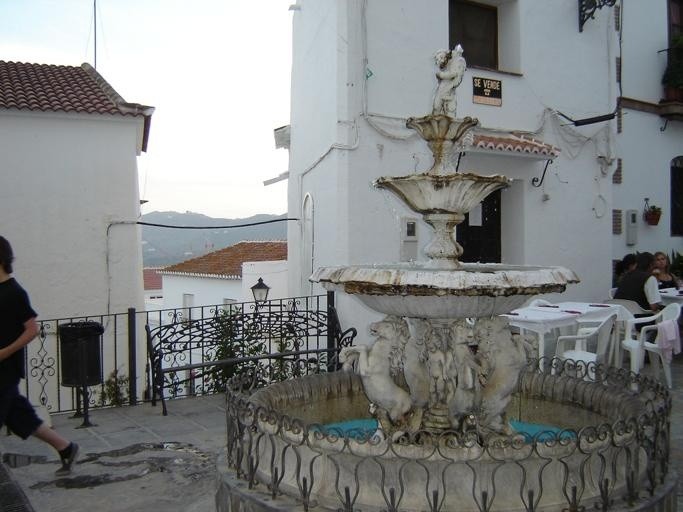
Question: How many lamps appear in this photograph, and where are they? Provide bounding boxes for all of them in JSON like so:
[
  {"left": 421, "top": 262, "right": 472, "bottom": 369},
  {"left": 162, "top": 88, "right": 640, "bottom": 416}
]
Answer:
[{"left": 250, "top": 277, "right": 287, "bottom": 307}]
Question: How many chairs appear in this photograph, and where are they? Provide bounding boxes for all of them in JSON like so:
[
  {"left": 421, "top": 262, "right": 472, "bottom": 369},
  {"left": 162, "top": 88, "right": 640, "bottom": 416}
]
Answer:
[{"left": 465, "top": 288, "right": 683, "bottom": 399}]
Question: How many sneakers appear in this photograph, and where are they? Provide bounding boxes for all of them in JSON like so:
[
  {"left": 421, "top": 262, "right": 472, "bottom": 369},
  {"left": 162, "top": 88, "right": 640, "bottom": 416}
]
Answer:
[{"left": 56, "top": 442, "right": 81, "bottom": 476}]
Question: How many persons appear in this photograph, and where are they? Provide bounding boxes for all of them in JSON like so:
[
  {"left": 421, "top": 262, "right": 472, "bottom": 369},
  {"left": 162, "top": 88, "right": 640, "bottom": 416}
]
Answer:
[
  {"left": 614, "top": 253, "right": 637, "bottom": 288},
  {"left": 651, "top": 251, "right": 680, "bottom": 289},
  {"left": 0, "top": 237, "right": 79, "bottom": 477},
  {"left": 614, "top": 251, "right": 661, "bottom": 334},
  {"left": 424, "top": 336, "right": 450, "bottom": 403},
  {"left": 429, "top": 42, "right": 466, "bottom": 117}
]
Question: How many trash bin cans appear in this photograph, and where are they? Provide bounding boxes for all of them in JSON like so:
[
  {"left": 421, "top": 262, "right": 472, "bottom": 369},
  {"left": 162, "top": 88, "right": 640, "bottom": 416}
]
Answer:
[{"left": 58, "top": 322, "right": 104, "bottom": 429}]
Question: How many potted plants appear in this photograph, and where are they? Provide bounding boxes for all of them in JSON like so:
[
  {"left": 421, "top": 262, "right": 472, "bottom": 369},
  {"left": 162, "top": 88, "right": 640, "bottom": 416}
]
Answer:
[{"left": 645, "top": 205, "right": 664, "bottom": 225}]
